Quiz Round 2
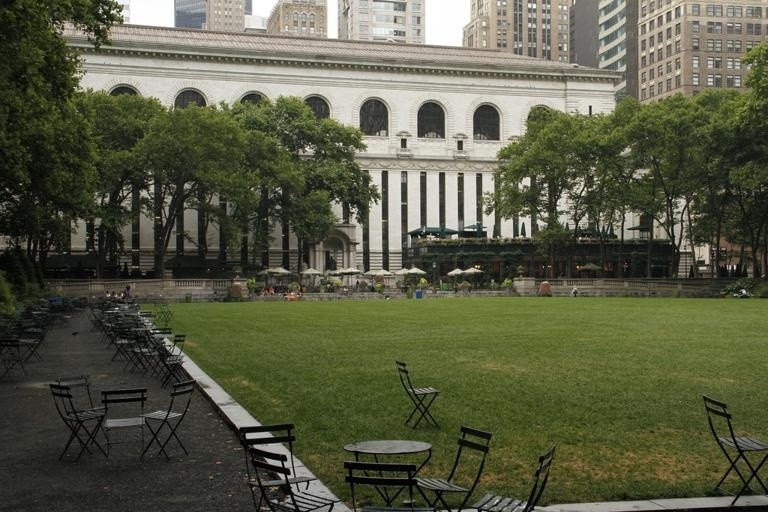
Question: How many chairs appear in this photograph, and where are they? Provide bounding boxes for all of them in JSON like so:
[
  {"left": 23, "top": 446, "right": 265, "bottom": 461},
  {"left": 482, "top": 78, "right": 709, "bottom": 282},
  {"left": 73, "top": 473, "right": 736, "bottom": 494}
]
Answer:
[
  {"left": 0, "top": 295, "right": 187, "bottom": 389},
  {"left": 394, "top": 358, "right": 440, "bottom": 428},
  {"left": 702, "top": 395, "right": 768, "bottom": 505},
  {"left": 49, "top": 373, "right": 195, "bottom": 463}
]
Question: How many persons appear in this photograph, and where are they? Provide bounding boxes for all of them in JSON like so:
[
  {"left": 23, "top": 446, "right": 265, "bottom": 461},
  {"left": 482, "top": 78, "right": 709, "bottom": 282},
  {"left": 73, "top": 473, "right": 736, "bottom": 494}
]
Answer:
[
  {"left": 106, "top": 285, "right": 135, "bottom": 297},
  {"left": 269, "top": 286, "right": 275, "bottom": 296},
  {"left": 331, "top": 282, "right": 337, "bottom": 289},
  {"left": 327, "top": 282, "right": 331, "bottom": 289},
  {"left": 261, "top": 287, "right": 268, "bottom": 296},
  {"left": 301, "top": 285, "right": 307, "bottom": 292},
  {"left": 370, "top": 276, "right": 376, "bottom": 291}
]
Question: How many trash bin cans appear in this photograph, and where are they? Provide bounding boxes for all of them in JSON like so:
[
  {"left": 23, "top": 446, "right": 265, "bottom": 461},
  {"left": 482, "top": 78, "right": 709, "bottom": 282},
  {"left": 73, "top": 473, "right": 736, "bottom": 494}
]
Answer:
[{"left": 416, "top": 290, "right": 422, "bottom": 298}]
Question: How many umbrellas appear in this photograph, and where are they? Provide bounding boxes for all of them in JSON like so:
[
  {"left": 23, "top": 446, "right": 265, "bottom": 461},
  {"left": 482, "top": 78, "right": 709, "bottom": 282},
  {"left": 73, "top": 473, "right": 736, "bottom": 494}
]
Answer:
[
  {"left": 446, "top": 268, "right": 464, "bottom": 289},
  {"left": 461, "top": 266, "right": 485, "bottom": 288},
  {"left": 257, "top": 266, "right": 427, "bottom": 286}
]
